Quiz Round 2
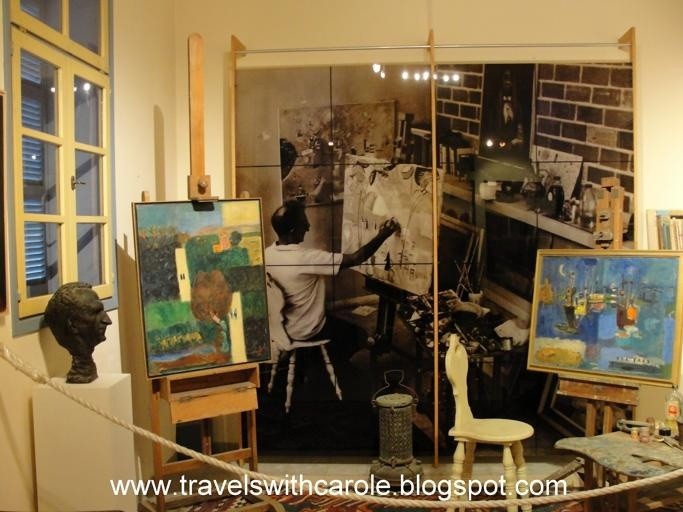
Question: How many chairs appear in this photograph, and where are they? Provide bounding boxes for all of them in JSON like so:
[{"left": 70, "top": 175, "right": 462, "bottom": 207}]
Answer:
[
  {"left": 264, "top": 272, "right": 343, "bottom": 414},
  {"left": 442, "top": 334, "right": 534, "bottom": 512}
]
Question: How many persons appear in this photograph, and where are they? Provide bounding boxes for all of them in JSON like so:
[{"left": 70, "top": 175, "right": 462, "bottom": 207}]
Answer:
[
  {"left": 264, "top": 200, "right": 396, "bottom": 390},
  {"left": 44, "top": 281, "right": 112, "bottom": 383}
]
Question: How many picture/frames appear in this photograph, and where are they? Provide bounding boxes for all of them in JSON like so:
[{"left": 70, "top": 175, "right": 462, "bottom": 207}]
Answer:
[{"left": 524, "top": 248, "right": 681, "bottom": 389}]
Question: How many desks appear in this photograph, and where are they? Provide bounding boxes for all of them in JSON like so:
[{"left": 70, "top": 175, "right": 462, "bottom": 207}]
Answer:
[{"left": 554, "top": 431, "right": 683, "bottom": 512}]
